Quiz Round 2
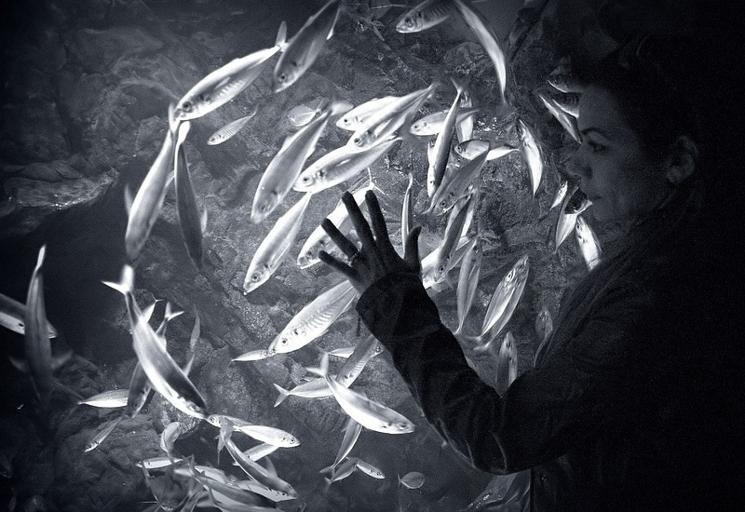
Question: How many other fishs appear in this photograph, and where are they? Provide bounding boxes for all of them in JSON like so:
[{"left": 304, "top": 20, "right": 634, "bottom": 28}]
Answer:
[{"left": 78, "top": 1, "right": 606, "bottom": 511}]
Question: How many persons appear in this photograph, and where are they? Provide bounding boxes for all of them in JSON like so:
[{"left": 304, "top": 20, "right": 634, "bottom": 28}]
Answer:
[{"left": 318, "top": 41, "right": 742, "bottom": 509}]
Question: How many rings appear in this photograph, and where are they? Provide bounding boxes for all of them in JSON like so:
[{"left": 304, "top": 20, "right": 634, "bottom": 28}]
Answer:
[{"left": 347, "top": 250, "right": 362, "bottom": 263}]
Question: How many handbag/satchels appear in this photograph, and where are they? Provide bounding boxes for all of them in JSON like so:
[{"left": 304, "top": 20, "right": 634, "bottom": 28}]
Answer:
[{"left": 467, "top": 469, "right": 531, "bottom": 512}]
